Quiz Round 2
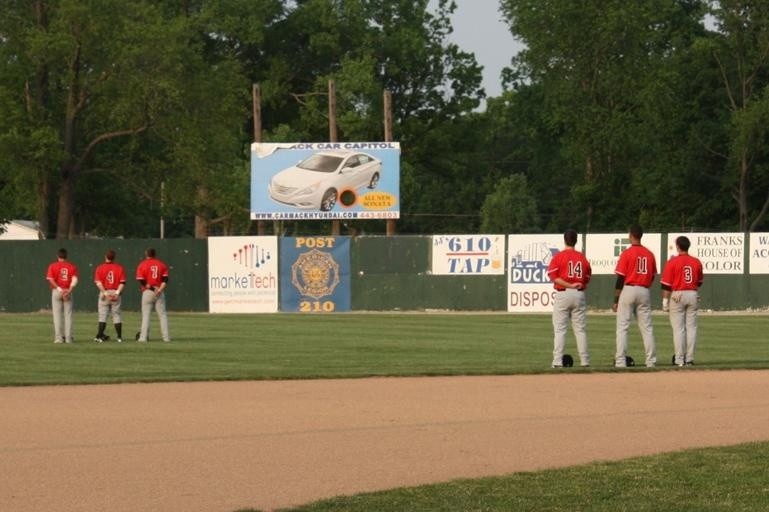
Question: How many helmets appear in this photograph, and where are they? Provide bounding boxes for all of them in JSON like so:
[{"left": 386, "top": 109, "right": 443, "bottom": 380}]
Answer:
[
  {"left": 136, "top": 332, "right": 149, "bottom": 342},
  {"left": 564, "top": 355, "right": 573, "bottom": 368},
  {"left": 615, "top": 357, "right": 635, "bottom": 367}
]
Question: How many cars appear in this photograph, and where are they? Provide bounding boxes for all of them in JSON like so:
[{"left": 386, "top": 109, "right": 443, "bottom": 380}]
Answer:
[{"left": 268, "top": 147, "right": 383, "bottom": 210}]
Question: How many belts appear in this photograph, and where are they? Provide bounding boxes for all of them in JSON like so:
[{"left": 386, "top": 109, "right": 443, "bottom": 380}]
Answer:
[
  {"left": 559, "top": 289, "right": 584, "bottom": 292},
  {"left": 627, "top": 284, "right": 649, "bottom": 289}
]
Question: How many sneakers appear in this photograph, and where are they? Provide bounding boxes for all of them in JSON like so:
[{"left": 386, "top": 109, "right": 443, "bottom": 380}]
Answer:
[{"left": 686, "top": 362, "right": 695, "bottom": 367}]
